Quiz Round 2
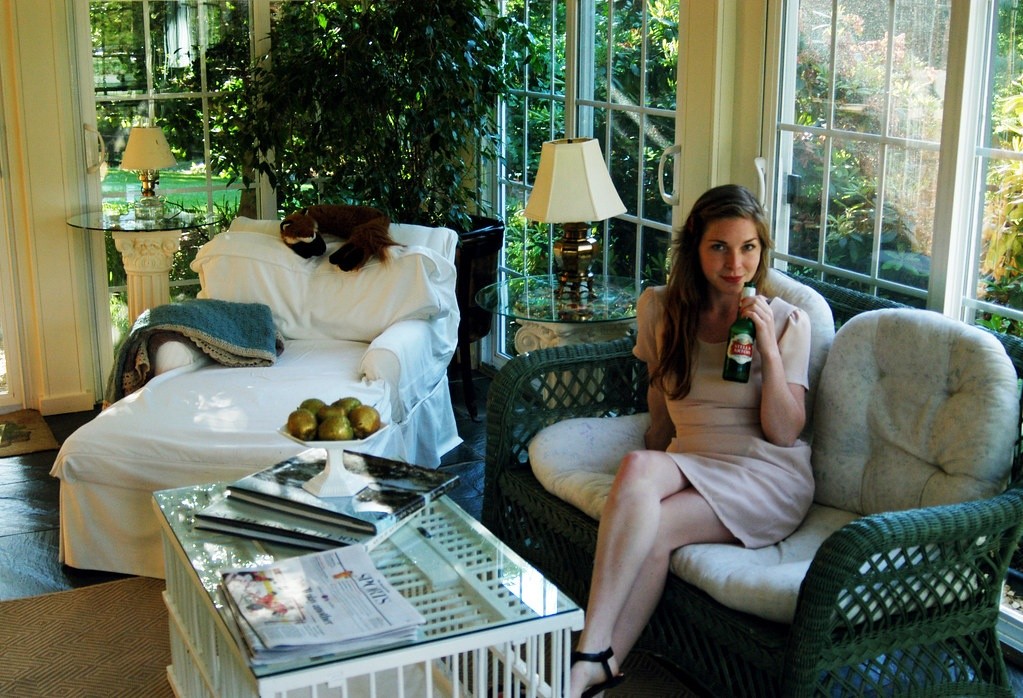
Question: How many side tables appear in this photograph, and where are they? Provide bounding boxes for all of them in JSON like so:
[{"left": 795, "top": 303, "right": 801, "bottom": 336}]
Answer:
[{"left": 65, "top": 208, "right": 224, "bottom": 326}]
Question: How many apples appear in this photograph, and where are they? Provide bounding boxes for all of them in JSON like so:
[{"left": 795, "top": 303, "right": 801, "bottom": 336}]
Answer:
[{"left": 288, "top": 396, "right": 381, "bottom": 441}]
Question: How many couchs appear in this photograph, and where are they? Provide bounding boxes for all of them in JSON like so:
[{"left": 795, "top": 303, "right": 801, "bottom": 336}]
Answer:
[{"left": 48, "top": 214, "right": 464, "bottom": 578}]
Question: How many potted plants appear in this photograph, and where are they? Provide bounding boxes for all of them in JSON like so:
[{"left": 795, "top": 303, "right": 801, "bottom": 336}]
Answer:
[{"left": 210, "top": 0, "right": 552, "bottom": 422}]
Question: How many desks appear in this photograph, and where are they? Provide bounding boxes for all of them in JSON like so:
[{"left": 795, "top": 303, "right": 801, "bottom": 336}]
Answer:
[
  {"left": 475, "top": 273, "right": 660, "bottom": 426},
  {"left": 152, "top": 479, "right": 584, "bottom": 698}
]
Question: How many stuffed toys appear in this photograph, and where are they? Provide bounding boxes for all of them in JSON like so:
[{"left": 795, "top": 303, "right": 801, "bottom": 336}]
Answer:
[{"left": 279, "top": 204, "right": 396, "bottom": 271}]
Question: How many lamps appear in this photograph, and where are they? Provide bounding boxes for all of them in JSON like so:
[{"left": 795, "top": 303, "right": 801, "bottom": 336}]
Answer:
[
  {"left": 118, "top": 117, "right": 177, "bottom": 221},
  {"left": 521, "top": 135, "right": 628, "bottom": 303}
]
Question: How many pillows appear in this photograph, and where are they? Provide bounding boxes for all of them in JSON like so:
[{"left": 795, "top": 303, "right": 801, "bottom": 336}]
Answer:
[{"left": 189, "top": 230, "right": 450, "bottom": 341}]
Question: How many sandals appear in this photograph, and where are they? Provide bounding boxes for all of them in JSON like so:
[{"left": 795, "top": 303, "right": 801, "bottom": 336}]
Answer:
[{"left": 559, "top": 645, "right": 630, "bottom": 698}]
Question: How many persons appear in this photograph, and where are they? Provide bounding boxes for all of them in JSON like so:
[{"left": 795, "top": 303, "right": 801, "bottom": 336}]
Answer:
[{"left": 570, "top": 182, "right": 815, "bottom": 698}]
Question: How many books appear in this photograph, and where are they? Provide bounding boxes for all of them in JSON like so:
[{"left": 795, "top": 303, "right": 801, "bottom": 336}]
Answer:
[{"left": 195, "top": 445, "right": 460, "bottom": 554}]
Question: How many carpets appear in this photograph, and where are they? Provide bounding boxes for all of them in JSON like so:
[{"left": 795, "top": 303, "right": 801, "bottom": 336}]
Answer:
[
  {"left": 0, "top": 576, "right": 700, "bottom": 698},
  {"left": 0, "top": 407, "right": 61, "bottom": 457}
]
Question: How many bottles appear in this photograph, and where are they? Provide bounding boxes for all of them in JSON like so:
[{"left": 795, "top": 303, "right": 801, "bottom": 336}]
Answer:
[{"left": 722, "top": 287, "right": 756, "bottom": 387}]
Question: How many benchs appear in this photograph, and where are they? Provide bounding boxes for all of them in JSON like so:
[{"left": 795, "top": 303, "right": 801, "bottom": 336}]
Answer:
[{"left": 480, "top": 265, "right": 1023, "bottom": 698}]
